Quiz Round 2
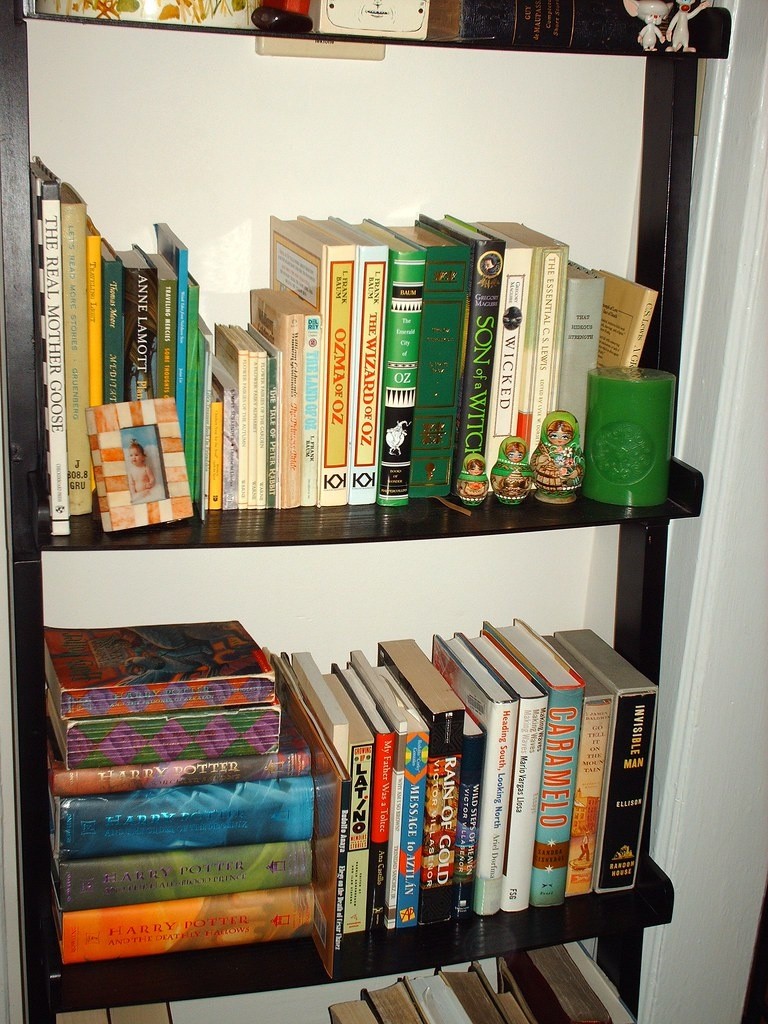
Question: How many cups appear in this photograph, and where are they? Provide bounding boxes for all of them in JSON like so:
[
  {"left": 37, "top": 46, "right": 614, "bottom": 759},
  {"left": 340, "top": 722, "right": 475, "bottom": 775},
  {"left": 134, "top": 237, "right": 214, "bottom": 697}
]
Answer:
[{"left": 584, "top": 365, "right": 677, "bottom": 506}]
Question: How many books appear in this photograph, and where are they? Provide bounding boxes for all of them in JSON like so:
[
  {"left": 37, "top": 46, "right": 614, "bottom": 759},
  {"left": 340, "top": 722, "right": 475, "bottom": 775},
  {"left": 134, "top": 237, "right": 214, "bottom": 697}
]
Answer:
[{"left": 30, "top": 156, "right": 659, "bottom": 1024}]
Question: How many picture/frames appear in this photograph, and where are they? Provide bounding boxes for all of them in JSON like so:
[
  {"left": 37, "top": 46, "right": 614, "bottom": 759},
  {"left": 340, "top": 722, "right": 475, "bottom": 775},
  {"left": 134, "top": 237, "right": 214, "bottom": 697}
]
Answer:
[{"left": 86, "top": 398, "right": 192, "bottom": 532}]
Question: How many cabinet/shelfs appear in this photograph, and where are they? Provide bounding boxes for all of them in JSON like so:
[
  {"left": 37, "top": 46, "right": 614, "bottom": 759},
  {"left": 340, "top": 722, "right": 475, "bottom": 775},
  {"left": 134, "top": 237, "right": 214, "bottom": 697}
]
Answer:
[{"left": 0, "top": 0, "right": 734, "bottom": 1024}]
